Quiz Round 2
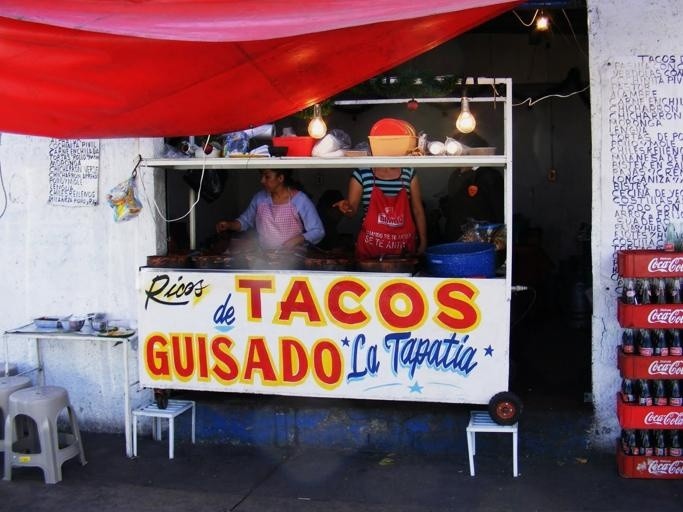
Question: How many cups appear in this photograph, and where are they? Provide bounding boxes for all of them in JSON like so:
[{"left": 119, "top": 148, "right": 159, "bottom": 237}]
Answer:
[{"left": 427, "top": 140, "right": 468, "bottom": 155}]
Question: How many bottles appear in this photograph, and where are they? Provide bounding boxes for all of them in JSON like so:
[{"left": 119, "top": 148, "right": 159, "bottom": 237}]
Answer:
[
  {"left": 663, "top": 224, "right": 676, "bottom": 253},
  {"left": 621, "top": 276, "right": 683, "bottom": 305},
  {"left": 619, "top": 327, "right": 682, "bottom": 356},
  {"left": 619, "top": 427, "right": 683, "bottom": 458},
  {"left": 621, "top": 378, "right": 682, "bottom": 407}
]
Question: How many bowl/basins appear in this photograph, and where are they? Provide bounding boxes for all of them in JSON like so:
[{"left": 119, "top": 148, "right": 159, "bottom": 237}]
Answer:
[
  {"left": 60, "top": 318, "right": 84, "bottom": 332},
  {"left": 92, "top": 320, "right": 106, "bottom": 332}
]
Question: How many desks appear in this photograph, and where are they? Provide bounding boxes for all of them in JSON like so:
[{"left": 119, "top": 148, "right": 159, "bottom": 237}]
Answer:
[{"left": 2, "top": 322, "right": 138, "bottom": 457}]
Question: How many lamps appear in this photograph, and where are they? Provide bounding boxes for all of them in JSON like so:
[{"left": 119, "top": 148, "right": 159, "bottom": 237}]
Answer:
[
  {"left": 308, "top": 103, "right": 328, "bottom": 141},
  {"left": 455, "top": 94, "right": 477, "bottom": 133},
  {"left": 536, "top": 8, "right": 550, "bottom": 34},
  {"left": 307, "top": 106, "right": 327, "bottom": 138}
]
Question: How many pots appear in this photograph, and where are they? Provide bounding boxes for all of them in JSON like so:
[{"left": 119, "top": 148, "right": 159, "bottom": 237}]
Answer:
[{"left": 146, "top": 247, "right": 427, "bottom": 275}]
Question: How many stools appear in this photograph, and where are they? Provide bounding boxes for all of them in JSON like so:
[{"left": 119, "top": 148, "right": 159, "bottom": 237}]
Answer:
[
  {"left": 0, "top": 363, "right": 87, "bottom": 485},
  {"left": 466, "top": 412, "right": 519, "bottom": 478},
  {"left": 132, "top": 399, "right": 195, "bottom": 460}
]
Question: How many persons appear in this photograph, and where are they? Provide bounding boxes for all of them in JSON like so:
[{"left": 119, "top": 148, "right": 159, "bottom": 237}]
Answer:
[
  {"left": 332, "top": 166, "right": 426, "bottom": 259},
  {"left": 433, "top": 128, "right": 506, "bottom": 271},
  {"left": 215, "top": 169, "right": 326, "bottom": 252}
]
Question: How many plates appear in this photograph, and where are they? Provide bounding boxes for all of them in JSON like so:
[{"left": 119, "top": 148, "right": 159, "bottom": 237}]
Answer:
[
  {"left": 368, "top": 116, "right": 417, "bottom": 137},
  {"left": 466, "top": 147, "right": 495, "bottom": 155},
  {"left": 33, "top": 318, "right": 60, "bottom": 329},
  {"left": 96, "top": 326, "right": 136, "bottom": 338}
]
